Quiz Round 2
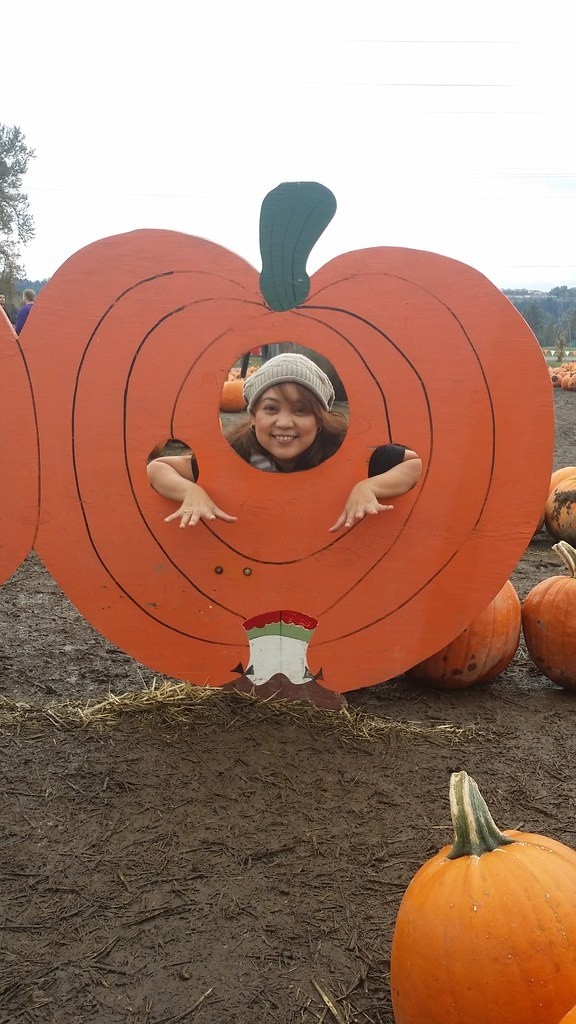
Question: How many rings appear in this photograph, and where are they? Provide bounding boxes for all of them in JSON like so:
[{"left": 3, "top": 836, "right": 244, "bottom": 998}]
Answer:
[{"left": 183, "top": 510, "right": 188, "bottom": 513}]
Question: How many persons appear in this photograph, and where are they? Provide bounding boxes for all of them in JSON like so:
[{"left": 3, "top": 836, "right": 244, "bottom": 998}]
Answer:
[
  {"left": 0, "top": 292, "right": 15, "bottom": 325},
  {"left": 147, "top": 353, "right": 423, "bottom": 532},
  {"left": 15, "top": 289, "right": 36, "bottom": 336}
]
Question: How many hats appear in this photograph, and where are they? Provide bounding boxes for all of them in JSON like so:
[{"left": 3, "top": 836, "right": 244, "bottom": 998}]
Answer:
[{"left": 242, "top": 353, "right": 335, "bottom": 413}]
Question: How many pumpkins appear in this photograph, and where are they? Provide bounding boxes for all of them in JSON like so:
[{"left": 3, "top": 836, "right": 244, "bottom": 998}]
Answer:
[
  {"left": 546, "top": 360, "right": 576, "bottom": 391},
  {"left": 389, "top": 771, "right": 576, "bottom": 1024},
  {"left": 148, "top": 366, "right": 257, "bottom": 458},
  {"left": 405, "top": 465, "right": 576, "bottom": 693}
]
